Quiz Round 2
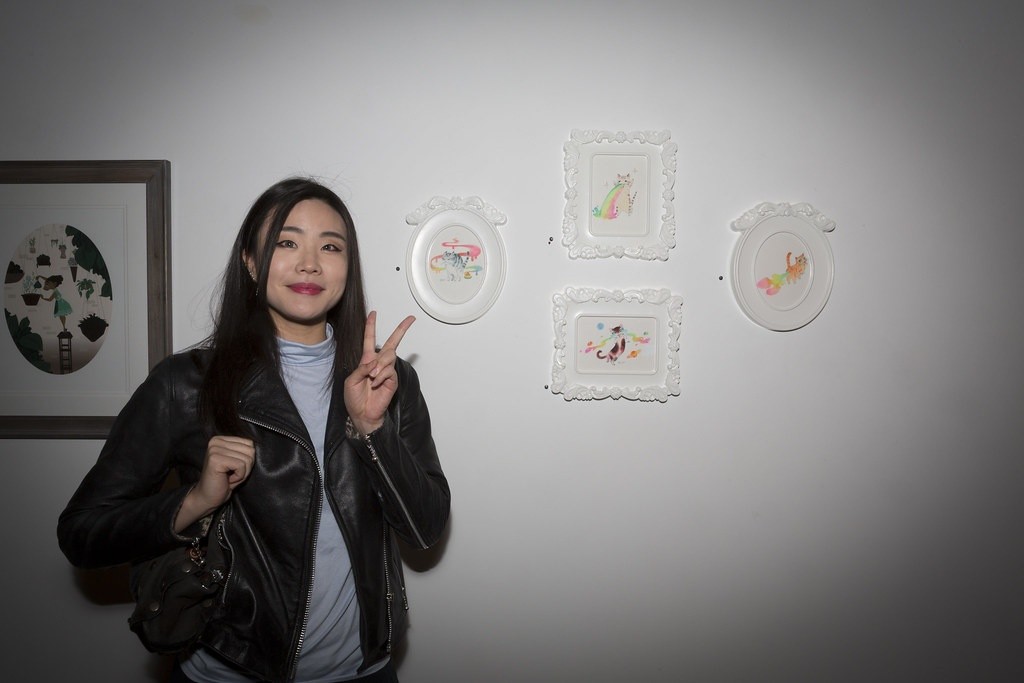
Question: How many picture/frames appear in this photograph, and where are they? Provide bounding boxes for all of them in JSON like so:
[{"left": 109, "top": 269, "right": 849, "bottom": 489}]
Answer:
[
  {"left": 406, "top": 196, "right": 506, "bottom": 325},
  {"left": 551, "top": 287, "right": 683, "bottom": 403},
  {"left": 0, "top": 159, "right": 172, "bottom": 440},
  {"left": 730, "top": 202, "right": 835, "bottom": 331},
  {"left": 562, "top": 128, "right": 677, "bottom": 261}
]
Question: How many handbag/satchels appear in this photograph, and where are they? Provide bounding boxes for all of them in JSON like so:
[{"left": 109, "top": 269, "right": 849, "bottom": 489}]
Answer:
[{"left": 128, "top": 350, "right": 224, "bottom": 655}]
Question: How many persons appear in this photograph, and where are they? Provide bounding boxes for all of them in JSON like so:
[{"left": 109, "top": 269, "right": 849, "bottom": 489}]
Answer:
[{"left": 55, "top": 177, "right": 452, "bottom": 683}]
